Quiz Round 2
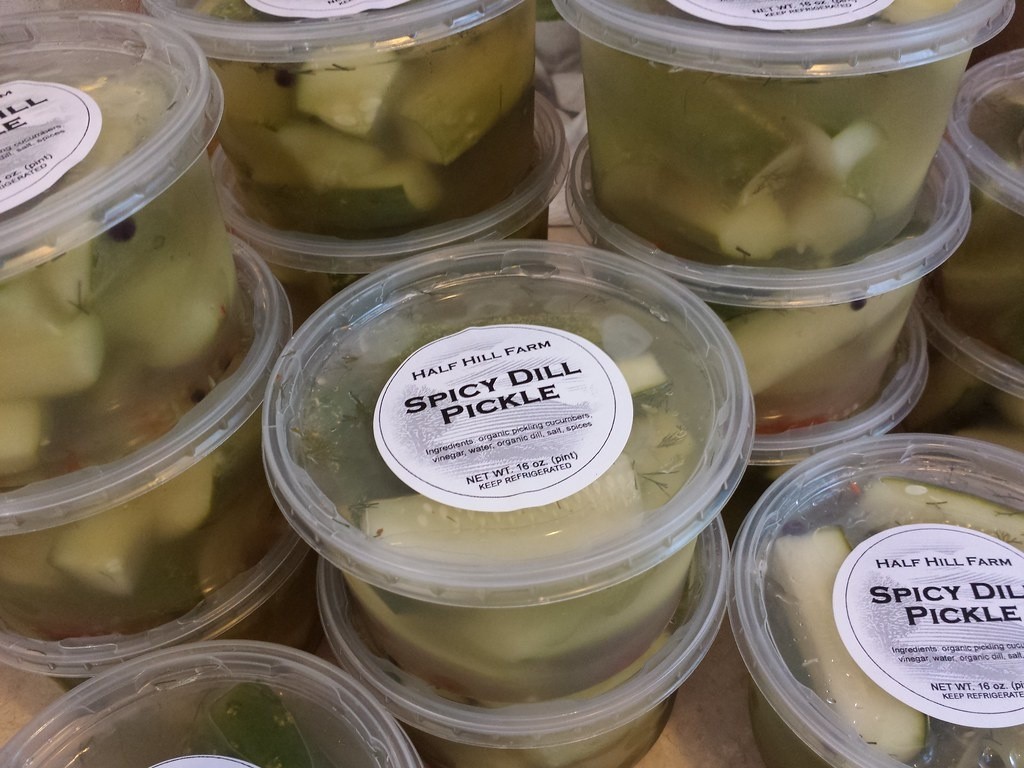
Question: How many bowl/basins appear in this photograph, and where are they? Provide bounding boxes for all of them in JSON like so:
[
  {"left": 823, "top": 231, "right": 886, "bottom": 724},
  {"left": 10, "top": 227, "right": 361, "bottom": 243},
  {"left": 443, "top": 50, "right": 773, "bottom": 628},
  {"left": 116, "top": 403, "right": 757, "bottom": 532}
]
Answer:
[{"left": 1, "top": 0, "right": 1024, "bottom": 767}]
